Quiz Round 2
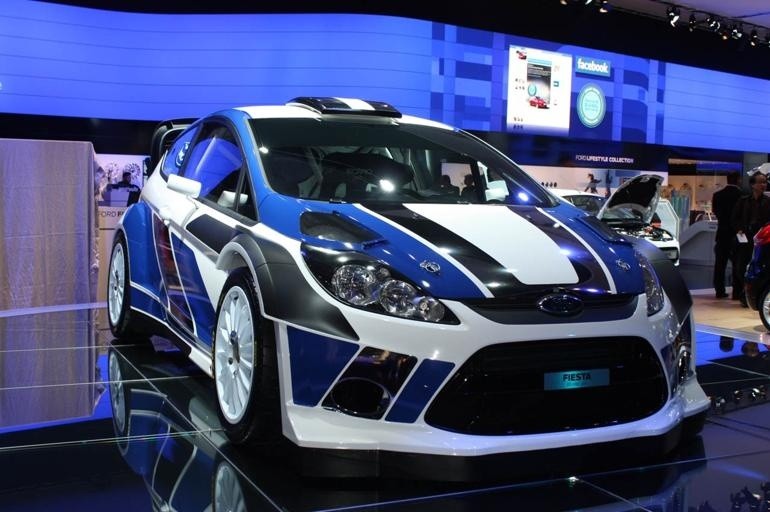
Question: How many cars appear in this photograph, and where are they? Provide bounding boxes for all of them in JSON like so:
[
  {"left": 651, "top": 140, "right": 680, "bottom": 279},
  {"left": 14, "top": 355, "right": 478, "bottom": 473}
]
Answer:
[
  {"left": 542, "top": 182, "right": 683, "bottom": 267},
  {"left": 743, "top": 225, "right": 769, "bottom": 333},
  {"left": 106, "top": 96, "right": 711, "bottom": 460}
]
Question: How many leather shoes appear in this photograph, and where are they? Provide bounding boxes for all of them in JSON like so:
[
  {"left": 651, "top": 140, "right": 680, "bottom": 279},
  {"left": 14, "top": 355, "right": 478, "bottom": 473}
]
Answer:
[{"left": 716, "top": 293, "right": 740, "bottom": 299}]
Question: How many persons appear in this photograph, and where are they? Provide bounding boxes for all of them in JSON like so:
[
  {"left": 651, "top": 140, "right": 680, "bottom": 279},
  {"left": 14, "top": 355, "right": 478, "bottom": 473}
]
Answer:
[
  {"left": 711, "top": 169, "right": 754, "bottom": 300},
  {"left": 442, "top": 174, "right": 459, "bottom": 197},
  {"left": 732, "top": 171, "right": 770, "bottom": 307},
  {"left": 584, "top": 174, "right": 602, "bottom": 193},
  {"left": 120, "top": 172, "right": 141, "bottom": 205},
  {"left": 459, "top": 174, "right": 478, "bottom": 202}
]
